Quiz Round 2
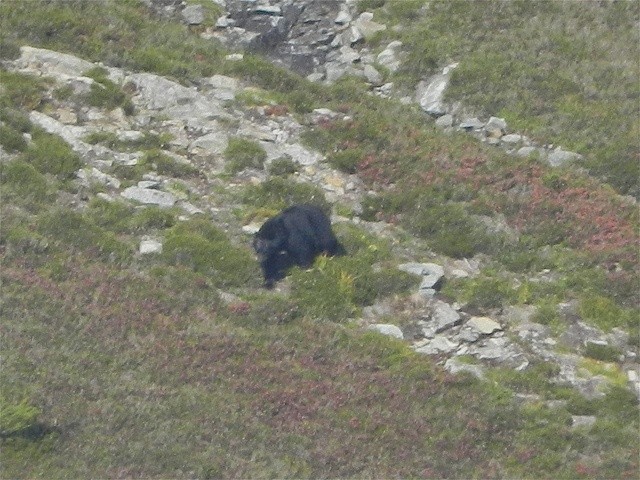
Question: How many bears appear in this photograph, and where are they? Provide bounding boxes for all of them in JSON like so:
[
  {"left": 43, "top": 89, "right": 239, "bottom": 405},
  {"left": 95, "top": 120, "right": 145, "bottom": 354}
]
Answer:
[{"left": 251, "top": 203, "right": 348, "bottom": 290}]
